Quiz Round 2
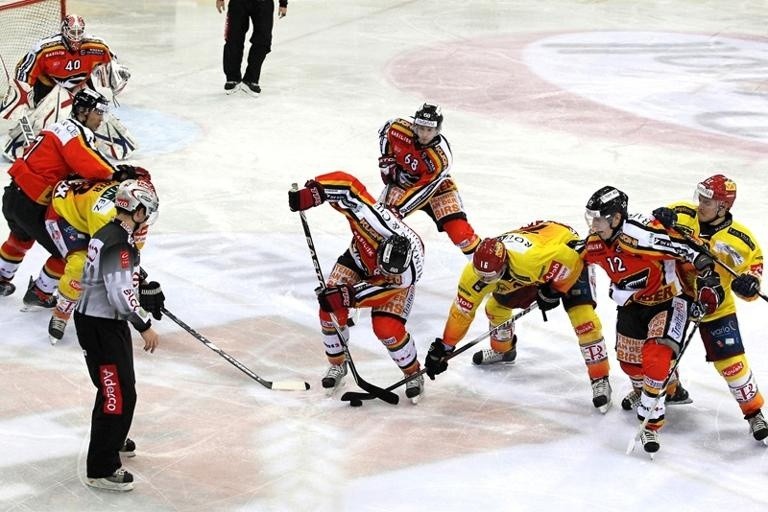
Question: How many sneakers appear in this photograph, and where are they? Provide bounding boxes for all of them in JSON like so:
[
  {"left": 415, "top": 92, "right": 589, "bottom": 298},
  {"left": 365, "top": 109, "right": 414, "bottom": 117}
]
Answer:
[
  {"left": 321, "top": 361, "right": 348, "bottom": 388},
  {"left": 224, "top": 81, "right": 261, "bottom": 93},
  {"left": 591, "top": 375, "right": 689, "bottom": 453},
  {"left": 87, "top": 468, "right": 134, "bottom": 482},
  {"left": 405, "top": 363, "right": 425, "bottom": 398},
  {"left": 0, "top": 274, "right": 16, "bottom": 296},
  {"left": 48, "top": 314, "right": 67, "bottom": 340},
  {"left": 744, "top": 409, "right": 768, "bottom": 441},
  {"left": 119, "top": 437, "right": 136, "bottom": 452}
]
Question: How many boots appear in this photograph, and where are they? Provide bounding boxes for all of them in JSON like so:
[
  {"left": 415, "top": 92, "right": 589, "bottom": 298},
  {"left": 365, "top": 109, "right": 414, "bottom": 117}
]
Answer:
[
  {"left": 472, "top": 334, "right": 518, "bottom": 365},
  {"left": 23, "top": 275, "right": 57, "bottom": 309}
]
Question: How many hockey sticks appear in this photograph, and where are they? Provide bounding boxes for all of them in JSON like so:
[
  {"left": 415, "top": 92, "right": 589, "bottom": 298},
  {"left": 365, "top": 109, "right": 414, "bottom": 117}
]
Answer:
[
  {"left": 292, "top": 183, "right": 399, "bottom": 404},
  {"left": 626, "top": 303, "right": 709, "bottom": 455},
  {"left": 341, "top": 304, "right": 539, "bottom": 401},
  {"left": 161, "top": 308, "right": 310, "bottom": 392},
  {"left": 347, "top": 307, "right": 360, "bottom": 327}
]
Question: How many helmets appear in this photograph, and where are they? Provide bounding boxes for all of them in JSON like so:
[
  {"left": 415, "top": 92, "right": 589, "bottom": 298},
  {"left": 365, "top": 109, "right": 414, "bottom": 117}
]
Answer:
[
  {"left": 413, "top": 103, "right": 444, "bottom": 128},
  {"left": 697, "top": 174, "right": 737, "bottom": 210},
  {"left": 71, "top": 87, "right": 110, "bottom": 117},
  {"left": 584, "top": 186, "right": 629, "bottom": 220},
  {"left": 471, "top": 237, "right": 509, "bottom": 285},
  {"left": 60, "top": 14, "right": 87, "bottom": 52},
  {"left": 114, "top": 178, "right": 159, "bottom": 225},
  {"left": 377, "top": 235, "right": 413, "bottom": 278}
]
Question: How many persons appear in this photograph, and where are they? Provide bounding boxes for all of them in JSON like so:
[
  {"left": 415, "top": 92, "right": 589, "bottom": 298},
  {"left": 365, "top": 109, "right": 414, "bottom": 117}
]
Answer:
[
  {"left": 372, "top": 101, "right": 482, "bottom": 262},
  {"left": 567, "top": 185, "right": 725, "bottom": 452},
  {"left": 216, "top": 0, "right": 289, "bottom": 96},
  {"left": 425, "top": 219, "right": 612, "bottom": 408},
  {"left": 1, "top": 12, "right": 165, "bottom": 489},
  {"left": 651, "top": 174, "right": 767, "bottom": 443},
  {"left": 287, "top": 171, "right": 428, "bottom": 399}
]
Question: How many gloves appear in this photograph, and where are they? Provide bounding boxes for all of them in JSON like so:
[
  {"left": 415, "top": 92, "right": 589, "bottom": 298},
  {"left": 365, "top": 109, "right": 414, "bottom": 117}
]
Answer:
[
  {"left": 652, "top": 207, "right": 678, "bottom": 229},
  {"left": 424, "top": 337, "right": 455, "bottom": 380},
  {"left": 288, "top": 179, "right": 327, "bottom": 212},
  {"left": 139, "top": 281, "right": 165, "bottom": 320},
  {"left": 537, "top": 279, "right": 563, "bottom": 311},
  {"left": 731, "top": 273, "right": 760, "bottom": 297},
  {"left": 314, "top": 284, "right": 356, "bottom": 312},
  {"left": 697, "top": 276, "right": 726, "bottom": 315}
]
